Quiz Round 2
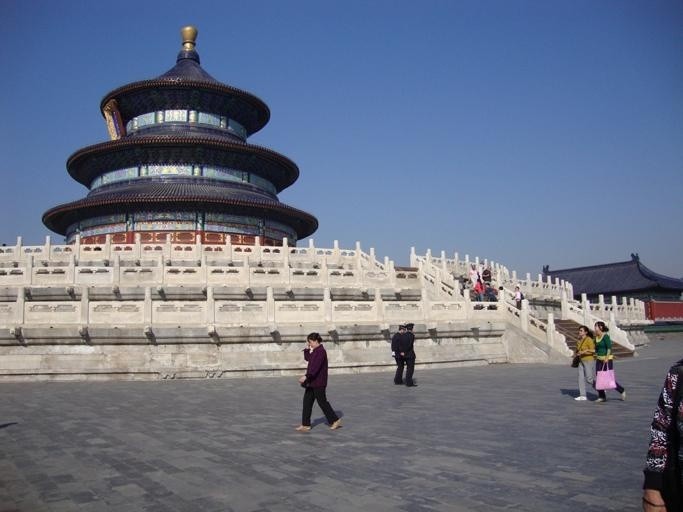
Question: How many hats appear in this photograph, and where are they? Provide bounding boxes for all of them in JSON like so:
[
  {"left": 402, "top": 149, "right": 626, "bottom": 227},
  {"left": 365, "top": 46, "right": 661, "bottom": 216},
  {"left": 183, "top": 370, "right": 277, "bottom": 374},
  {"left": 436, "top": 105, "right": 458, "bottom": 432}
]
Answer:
[{"left": 397, "top": 322, "right": 415, "bottom": 329}]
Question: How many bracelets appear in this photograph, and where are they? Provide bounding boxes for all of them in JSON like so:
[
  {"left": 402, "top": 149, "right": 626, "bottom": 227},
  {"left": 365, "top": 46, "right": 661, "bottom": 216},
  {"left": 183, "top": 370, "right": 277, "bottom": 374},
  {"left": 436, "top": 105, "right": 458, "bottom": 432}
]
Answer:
[{"left": 641, "top": 495, "right": 667, "bottom": 507}]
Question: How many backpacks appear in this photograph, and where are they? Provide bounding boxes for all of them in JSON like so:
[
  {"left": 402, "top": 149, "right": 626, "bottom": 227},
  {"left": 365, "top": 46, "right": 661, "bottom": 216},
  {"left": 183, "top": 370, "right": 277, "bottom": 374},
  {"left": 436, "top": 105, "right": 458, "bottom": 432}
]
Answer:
[{"left": 518, "top": 292, "right": 524, "bottom": 301}]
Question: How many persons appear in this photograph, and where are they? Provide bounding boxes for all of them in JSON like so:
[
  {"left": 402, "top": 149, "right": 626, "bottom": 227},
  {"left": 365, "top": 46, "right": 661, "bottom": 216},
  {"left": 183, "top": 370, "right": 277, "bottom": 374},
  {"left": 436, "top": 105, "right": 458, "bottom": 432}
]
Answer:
[
  {"left": 580, "top": 322, "right": 627, "bottom": 403},
  {"left": 571, "top": 326, "right": 596, "bottom": 402},
  {"left": 641, "top": 357, "right": 683, "bottom": 512},
  {"left": 461, "top": 264, "right": 524, "bottom": 317},
  {"left": 398, "top": 321, "right": 418, "bottom": 387},
  {"left": 292, "top": 332, "right": 342, "bottom": 432},
  {"left": 389, "top": 324, "right": 408, "bottom": 385}
]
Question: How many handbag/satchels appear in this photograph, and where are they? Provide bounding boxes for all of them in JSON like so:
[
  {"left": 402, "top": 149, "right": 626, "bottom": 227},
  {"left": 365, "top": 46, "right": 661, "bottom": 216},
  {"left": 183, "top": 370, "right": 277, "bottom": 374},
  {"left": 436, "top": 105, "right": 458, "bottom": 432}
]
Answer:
[
  {"left": 595, "top": 361, "right": 616, "bottom": 391},
  {"left": 571, "top": 353, "right": 579, "bottom": 369}
]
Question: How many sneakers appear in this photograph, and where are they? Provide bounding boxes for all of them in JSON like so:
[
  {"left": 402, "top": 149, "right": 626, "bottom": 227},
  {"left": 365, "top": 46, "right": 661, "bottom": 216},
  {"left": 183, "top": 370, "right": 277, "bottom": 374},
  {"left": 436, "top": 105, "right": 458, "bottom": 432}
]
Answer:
[
  {"left": 294, "top": 426, "right": 311, "bottom": 431},
  {"left": 330, "top": 417, "right": 341, "bottom": 429},
  {"left": 574, "top": 394, "right": 586, "bottom": 401},
  {"left": 392, "top": 378, "right": 417, "bottom": 387},
  {"left": 618, "top": 387, "right": 626, "bottom": 401},
  {"left": 594, "top": 398, "right": 605, "bottom": 403}
]
